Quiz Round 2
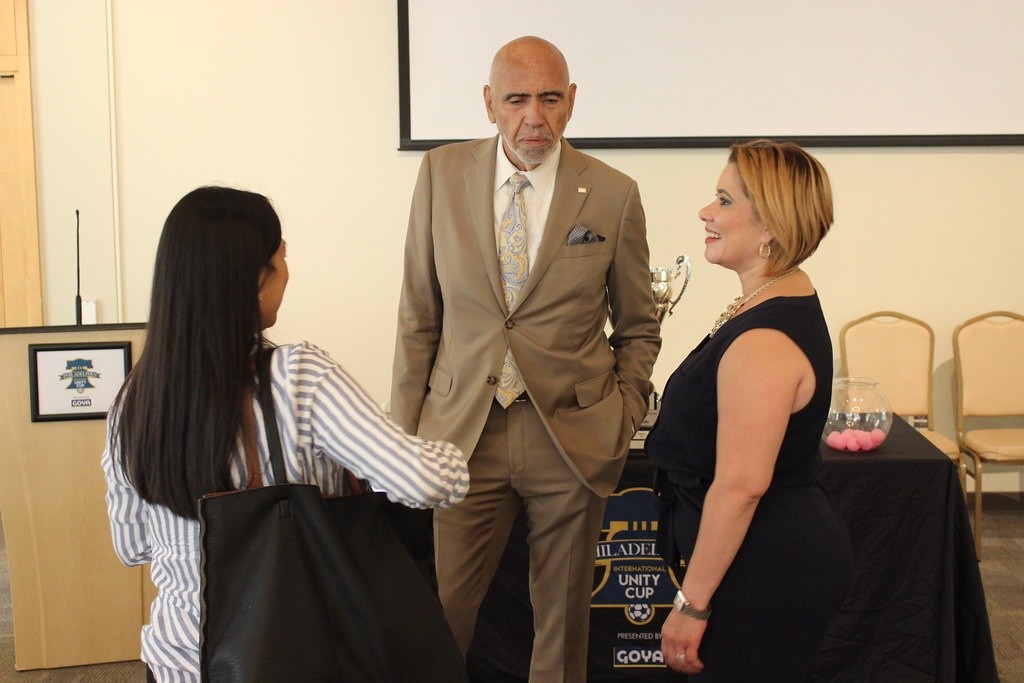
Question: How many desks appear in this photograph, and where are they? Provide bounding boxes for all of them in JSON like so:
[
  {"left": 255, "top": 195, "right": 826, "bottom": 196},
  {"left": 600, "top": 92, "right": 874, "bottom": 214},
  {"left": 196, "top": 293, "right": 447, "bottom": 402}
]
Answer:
[{"left": 383, "top": 412, "right": 1000, "bottom": 683}]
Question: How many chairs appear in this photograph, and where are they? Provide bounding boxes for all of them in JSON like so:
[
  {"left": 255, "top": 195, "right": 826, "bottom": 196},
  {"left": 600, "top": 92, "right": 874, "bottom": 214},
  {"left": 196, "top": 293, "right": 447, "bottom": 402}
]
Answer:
[
  {"left": 839, "top": 310, "right": 968, "bottom": 498},
  {"left": 953, "top": 310, "right": 1024, "bottom": 561}
]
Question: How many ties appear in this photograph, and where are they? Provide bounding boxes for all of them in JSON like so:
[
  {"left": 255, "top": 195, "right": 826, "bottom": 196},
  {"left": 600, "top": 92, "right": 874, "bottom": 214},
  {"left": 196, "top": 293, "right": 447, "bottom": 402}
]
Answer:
[{"left": 494, "top": 174, "right": 530, "bottom": 410}]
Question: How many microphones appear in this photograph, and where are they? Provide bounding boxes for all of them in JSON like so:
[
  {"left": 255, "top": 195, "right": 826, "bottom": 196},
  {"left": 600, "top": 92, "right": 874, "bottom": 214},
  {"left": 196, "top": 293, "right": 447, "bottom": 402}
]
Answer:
[{"left": 74, "top": 209, "right": 82, "bottom": 325}]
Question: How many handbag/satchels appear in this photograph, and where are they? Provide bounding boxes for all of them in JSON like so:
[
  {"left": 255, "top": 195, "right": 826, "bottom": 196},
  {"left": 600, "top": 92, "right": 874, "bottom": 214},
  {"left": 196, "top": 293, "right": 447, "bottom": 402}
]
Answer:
[{"left": 199, "top": 346, "right": 468, "bottom": 683}]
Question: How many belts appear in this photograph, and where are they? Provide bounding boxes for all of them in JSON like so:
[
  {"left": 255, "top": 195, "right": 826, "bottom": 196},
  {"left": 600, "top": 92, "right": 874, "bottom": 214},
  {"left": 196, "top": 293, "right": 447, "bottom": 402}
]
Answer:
[{"left": 514, "top": 391, "right": 531, "bottom": 403}]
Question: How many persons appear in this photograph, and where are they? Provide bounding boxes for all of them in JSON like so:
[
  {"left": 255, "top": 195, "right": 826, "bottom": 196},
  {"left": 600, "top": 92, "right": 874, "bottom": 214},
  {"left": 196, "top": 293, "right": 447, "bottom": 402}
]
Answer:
[
  {"left": 389, "top": 35, "right": 662, "bottom": 683},
  {"left": 100, "top": 185, "right": 474, "bottom": 683},
  {"left": 643, "top": 138, "right": 838, "bottom": 683}
]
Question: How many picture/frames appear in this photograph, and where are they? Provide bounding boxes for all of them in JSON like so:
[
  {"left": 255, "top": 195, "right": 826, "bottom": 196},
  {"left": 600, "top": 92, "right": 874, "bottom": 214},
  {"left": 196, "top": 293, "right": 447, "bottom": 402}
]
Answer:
[{"left": 30, "top": 340, "right": 132, "bottom": 423}]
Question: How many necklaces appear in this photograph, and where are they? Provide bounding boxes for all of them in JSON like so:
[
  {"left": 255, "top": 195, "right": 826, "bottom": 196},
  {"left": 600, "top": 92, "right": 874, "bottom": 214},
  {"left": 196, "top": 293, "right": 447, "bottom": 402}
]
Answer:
[{"left": 709, "top": 267, "right": 799, "bottom": 339}]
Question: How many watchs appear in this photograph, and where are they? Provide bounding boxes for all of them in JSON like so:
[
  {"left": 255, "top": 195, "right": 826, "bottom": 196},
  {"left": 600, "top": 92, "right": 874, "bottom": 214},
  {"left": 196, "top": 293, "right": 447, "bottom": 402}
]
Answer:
[{"left": 673, "top": 591, "right": 712, "bottom": 620}]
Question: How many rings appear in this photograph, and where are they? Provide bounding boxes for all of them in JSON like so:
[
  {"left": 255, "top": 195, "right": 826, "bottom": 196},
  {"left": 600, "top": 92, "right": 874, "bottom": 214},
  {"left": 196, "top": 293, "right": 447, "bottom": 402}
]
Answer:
[{"left": 676, "top": 655, "right": 684, "bottom": 660}]
